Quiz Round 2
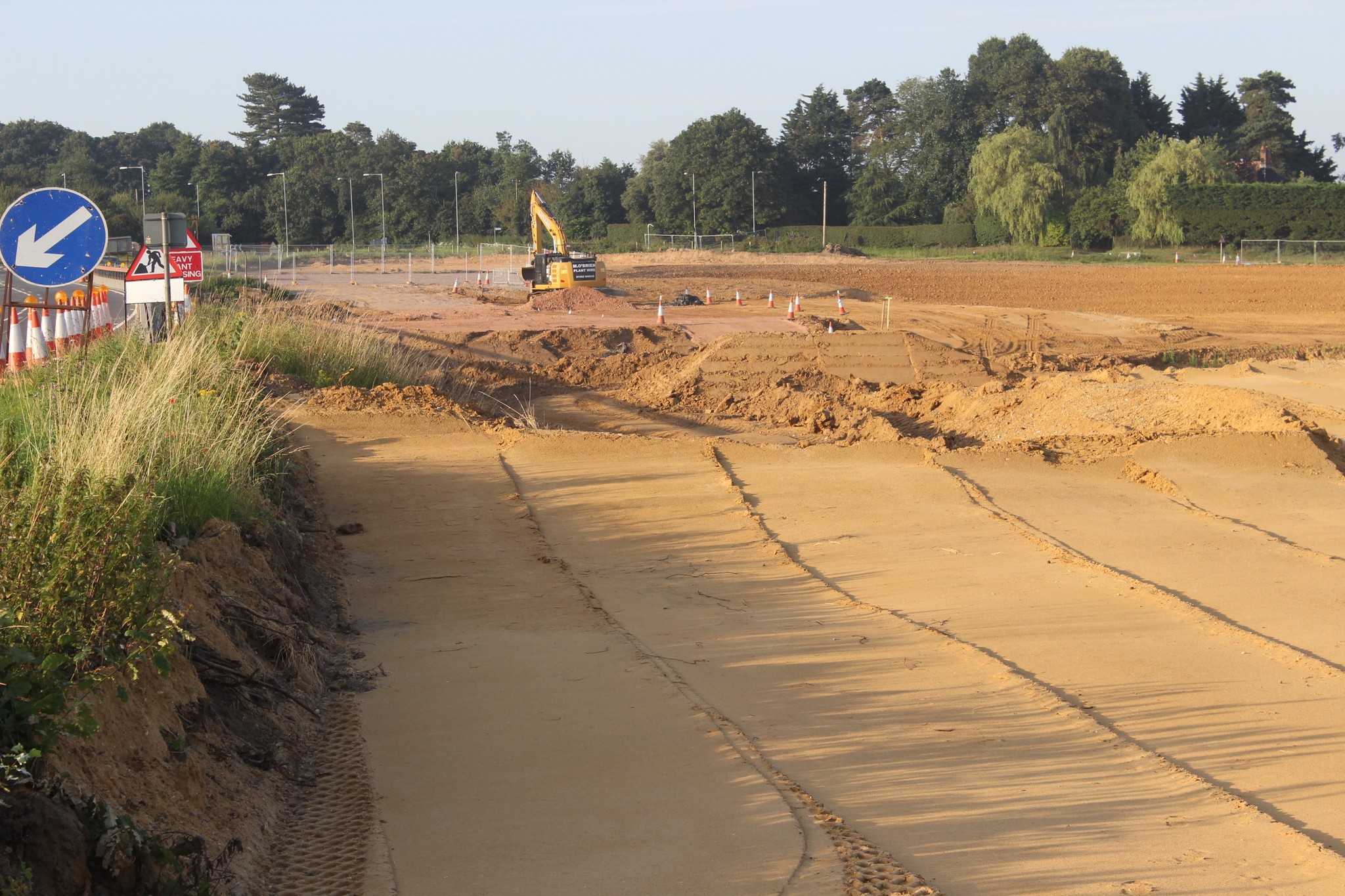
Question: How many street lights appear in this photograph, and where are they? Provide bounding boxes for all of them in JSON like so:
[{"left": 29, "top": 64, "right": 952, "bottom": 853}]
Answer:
[
  {"left": 60, "top": 173, "right": 67, "bottom": 187},
  {"left": 337, "top": 177, "right": 355, "bottom": 253},
  {"left": 455, "top": 171, "right": 461, "bottom": 254},
  {"left": 267, "top": 172, "right": 288, "bottom": 258},
  {"left": 120, "top": 165, "right": 145, "bottom": 217},
  {"left": 362, "top": 173, "right": 385, "bottom": 237},
  {"left": 684, "top": 172, "right": 695, "bottom": 250},
  {"left": 752, "top": 171, "right": 763, "bottom": 236},
  {"left": 187, "top": 182, "right": 200, "bottom": 221}
]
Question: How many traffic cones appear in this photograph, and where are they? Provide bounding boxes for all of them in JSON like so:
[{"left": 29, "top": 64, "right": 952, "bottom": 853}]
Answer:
[
  {"left": 1174, "top": 252, "right": 1180, "bottom": 263},
  {"left": 684, "top": 285, "right": 689, "bottom": 295},
  {"left": 794, "top": 291, "right": 801, "bottom": 311},
  {"left": 1127, "top": 251, "right": 1130, "bottom": 258},
  {"left": 828, "top": 322, "right": 833, "bottom": 334},
  {"left": 25, "top": 285, "right": 114, "bottom": 372},
  {"left": 735, "top": 288, "right": 743, "bottom": 307},
  {"left": 0, "top": 343, "right": 7, "bottom": 374},
  {"left": 476, "top": 270, "right": 482, "bottom": 285},
  {"left": 785, "top": 297, "right": 795, "bottom": 319},
  {"left": 837, "top": 290, "right": 849, "bottom": 315},
  {"left": 1222, "top": 253, "right": 1226, "bottom": 263},
  {"left": 1071, "top": 250, "right": 1074, "bottom": 258},
  {"left": 1235, "top": 253, "right": 1240, "bottom": 266},
  {"left": 484, "top": 270, "right": 490, "bottom": 285},
  {"left": 656, "top": 295, "right": 666, "bottom": 324},
  {"left": 451, "top": 277, "right": 458, "bottom": 292},
  {"left": 704, "top": 286, "right": 714, "bottom": 305},
  {"left": 9, "top": 306, "right": 28, "bottom": 375},
  {"left": 767, "top": 289, "right": 775, "bottom": 309}
]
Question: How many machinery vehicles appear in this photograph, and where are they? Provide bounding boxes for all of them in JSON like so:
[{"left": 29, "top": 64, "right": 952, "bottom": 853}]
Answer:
[{"left": 521, "top": 190, "right": 607, "bottom": 301}]
